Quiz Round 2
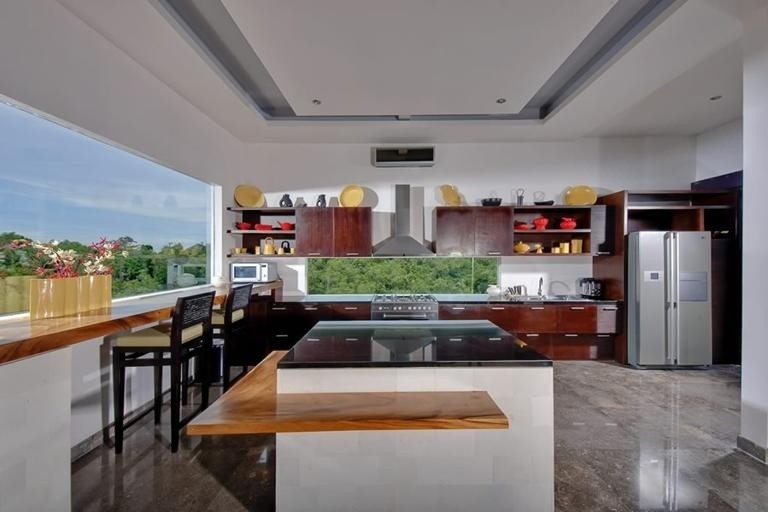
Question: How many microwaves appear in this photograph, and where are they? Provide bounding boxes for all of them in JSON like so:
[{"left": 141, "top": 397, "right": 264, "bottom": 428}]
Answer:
[{"left": 230, "top": 261, "right": 277, "bottom": 283}]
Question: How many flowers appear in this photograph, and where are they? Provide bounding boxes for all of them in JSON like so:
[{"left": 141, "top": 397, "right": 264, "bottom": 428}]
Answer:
[{"left": 7, "top": 234, "right": 130, "bottom": 278}]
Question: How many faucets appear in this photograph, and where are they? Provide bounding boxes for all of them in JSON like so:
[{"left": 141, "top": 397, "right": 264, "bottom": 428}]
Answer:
[{"left": 538, "top": 277, "right": 546, "bottom": 301}]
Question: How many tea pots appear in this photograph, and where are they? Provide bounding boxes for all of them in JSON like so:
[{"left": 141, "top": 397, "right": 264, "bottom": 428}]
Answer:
[{"left": 264, "top": 236, "right": 276, "bottom": 255}]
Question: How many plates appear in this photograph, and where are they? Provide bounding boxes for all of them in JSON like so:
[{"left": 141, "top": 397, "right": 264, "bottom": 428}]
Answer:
[
  {"left": 234, "top": 183, "right": 265, "bottom": 208},
  {"left": 339, "top": 185, "right": 364, "bottom": 207},
  {"left": 564, "top": 185, "right": 597, "bottom": 205}
]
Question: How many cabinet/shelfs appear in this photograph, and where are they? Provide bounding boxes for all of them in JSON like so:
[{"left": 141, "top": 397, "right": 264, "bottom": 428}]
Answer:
[
  {"left": 592, "top": 186, "right": 740, "bottom": 365},
  {"left": 226, "top": 207, "right": 296, "bottom": 257},
  {"left": 438, "top": 303, "right": 625, "bottom": 361},
  {"left": 297, "top": 207, "right": 373, "bottom": 256},
  {"left": 270, "top": 302, "right": 371, "bottom": 368},
  {"left": 432, "top": 205, "right": 616, "bottom": 255}
]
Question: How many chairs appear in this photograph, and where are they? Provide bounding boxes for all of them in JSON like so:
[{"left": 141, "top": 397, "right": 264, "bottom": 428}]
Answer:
[{"left": 108, "top": 281, "right": 253, "bottom": 454}]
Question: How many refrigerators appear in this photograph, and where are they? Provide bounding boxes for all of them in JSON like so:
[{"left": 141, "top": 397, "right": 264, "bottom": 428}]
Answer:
[{"left": 628, "top": 229, "right": 715, "bottom": 369}]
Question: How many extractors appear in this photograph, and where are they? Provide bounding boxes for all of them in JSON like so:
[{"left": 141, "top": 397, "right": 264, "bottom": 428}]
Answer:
[{"left": 374, "top": 184, "right": 435, "bottom": 256}]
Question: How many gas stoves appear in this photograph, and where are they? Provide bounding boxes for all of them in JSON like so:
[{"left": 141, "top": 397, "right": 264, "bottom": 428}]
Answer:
[{"left": 370, "top": 293, "right": 438, "bottom": 311}]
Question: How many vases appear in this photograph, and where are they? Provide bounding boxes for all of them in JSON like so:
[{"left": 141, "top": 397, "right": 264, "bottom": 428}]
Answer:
[{"left": 29, "top": 274, "right": 112, "bottom": 321}]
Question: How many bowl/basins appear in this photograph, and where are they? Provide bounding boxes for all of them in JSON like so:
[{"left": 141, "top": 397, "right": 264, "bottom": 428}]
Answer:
[
  {"left": 255, "top": 223, "right": 272, "bottom": 230},
  {"left": 236, "top": 222, "right": 251, "bottom": 230},
  {"left": 481, "top": 197, "right": 502, "bottom": 206},
  {"left": 280, "top": 222, "right": 294, "bottom": 230}
]
